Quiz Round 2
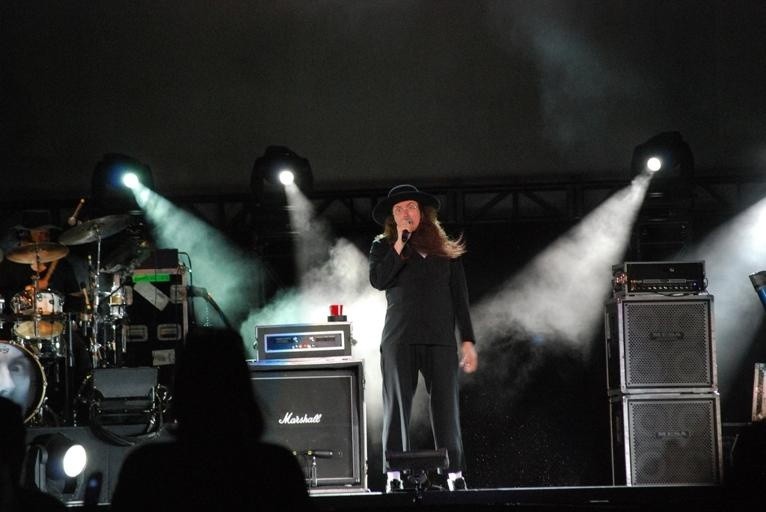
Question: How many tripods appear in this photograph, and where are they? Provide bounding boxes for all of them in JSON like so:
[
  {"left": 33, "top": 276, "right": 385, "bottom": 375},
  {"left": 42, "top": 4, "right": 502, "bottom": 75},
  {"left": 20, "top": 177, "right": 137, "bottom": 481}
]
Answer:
[
  {"left": 78, "top": 237, "right": 118, "bottom": 398},
  {"left": 26, "top": 236, "right": 73, "bottom": 428}
]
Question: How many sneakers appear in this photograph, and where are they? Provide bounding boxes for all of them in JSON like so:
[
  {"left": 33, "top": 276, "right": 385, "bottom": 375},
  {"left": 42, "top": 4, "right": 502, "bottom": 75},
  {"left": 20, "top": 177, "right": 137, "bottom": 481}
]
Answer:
[
  {"left": 447, "top": 476, "right": 468, "bottom": 491},
  {"left": 386, "top": 479, "right": 404, "bottom": 494}
]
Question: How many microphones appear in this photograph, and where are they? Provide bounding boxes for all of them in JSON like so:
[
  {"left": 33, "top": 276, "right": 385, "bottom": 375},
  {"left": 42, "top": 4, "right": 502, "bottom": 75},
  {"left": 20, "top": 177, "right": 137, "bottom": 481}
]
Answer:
[
  {"left": 68, "top": 198, "right": 85, "bottom": 225},
  {"left": 402, "top": 221, "right": 412, "bottom": 242},
  {"left": 81, "top": 281, "right": 92, "bottom": 310}
]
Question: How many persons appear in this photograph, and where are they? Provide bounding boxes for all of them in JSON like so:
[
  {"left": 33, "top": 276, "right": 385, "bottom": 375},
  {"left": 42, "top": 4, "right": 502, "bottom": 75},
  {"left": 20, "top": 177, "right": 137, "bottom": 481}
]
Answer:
[
  {"left": 368, "top": 184, "right": 480, "bottom": 490},
  {"left": 0, "top": 396, "right": 67, "bottom": 512},
  {"left": 1, "top": 224, "right": 112, "bottom": 317},
  {"left": 110, "top": 325, "right": 310, "bottom": 512},
  {"left": 721, "top": 413, "right": 766, "bottom": 512},
  {"left": 0, "top": 340, "right": 35, "bottom": 428}
]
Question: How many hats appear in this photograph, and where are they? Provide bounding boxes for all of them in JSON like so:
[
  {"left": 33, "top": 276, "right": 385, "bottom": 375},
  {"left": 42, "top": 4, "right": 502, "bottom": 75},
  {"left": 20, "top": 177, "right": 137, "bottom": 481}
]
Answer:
[{"left": 372, "top": 185, "right": 441, "bottom": 225}]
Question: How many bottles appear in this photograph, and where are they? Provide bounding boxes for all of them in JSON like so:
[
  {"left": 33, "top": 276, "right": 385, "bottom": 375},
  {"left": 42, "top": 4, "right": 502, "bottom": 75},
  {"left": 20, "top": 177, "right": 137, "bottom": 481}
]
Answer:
[
  {"left": 311, "top": 457, "right": 318, "bottom": 487},
  {"left": 108, "top": 273, "right": 124, "bottom": 317}
]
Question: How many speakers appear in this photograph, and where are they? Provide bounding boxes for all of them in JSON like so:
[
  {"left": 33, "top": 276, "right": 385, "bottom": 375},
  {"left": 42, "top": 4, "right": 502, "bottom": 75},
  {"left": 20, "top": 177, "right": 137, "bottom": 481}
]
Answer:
[
  {"left": 120, "top": 267, "right": 188, "bottom": 383},
  {"left": 604, "top": 295, "right": 718, "bottom": 395},
  {"left": 19, "top": 423, "right": 161, "bottom": 505},
  {"left": 609, "top": 392, "right": 724, "bottom": 487},
  {"left": 247, "top": 360, "right": 369, "bottom": 494}
]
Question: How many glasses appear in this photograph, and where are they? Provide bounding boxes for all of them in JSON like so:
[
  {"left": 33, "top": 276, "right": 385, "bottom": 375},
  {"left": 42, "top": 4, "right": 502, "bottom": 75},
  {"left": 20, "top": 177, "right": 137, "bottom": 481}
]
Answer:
[{"left": 392, "top": 204, "right": 419, "bottom": 215}]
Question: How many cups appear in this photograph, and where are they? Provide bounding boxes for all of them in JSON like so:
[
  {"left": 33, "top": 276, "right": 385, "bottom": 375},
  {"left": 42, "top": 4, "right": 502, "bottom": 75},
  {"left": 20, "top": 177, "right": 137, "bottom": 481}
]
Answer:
[{"left": 330, "top": 304, "right": 343, "bottom": 316}]
